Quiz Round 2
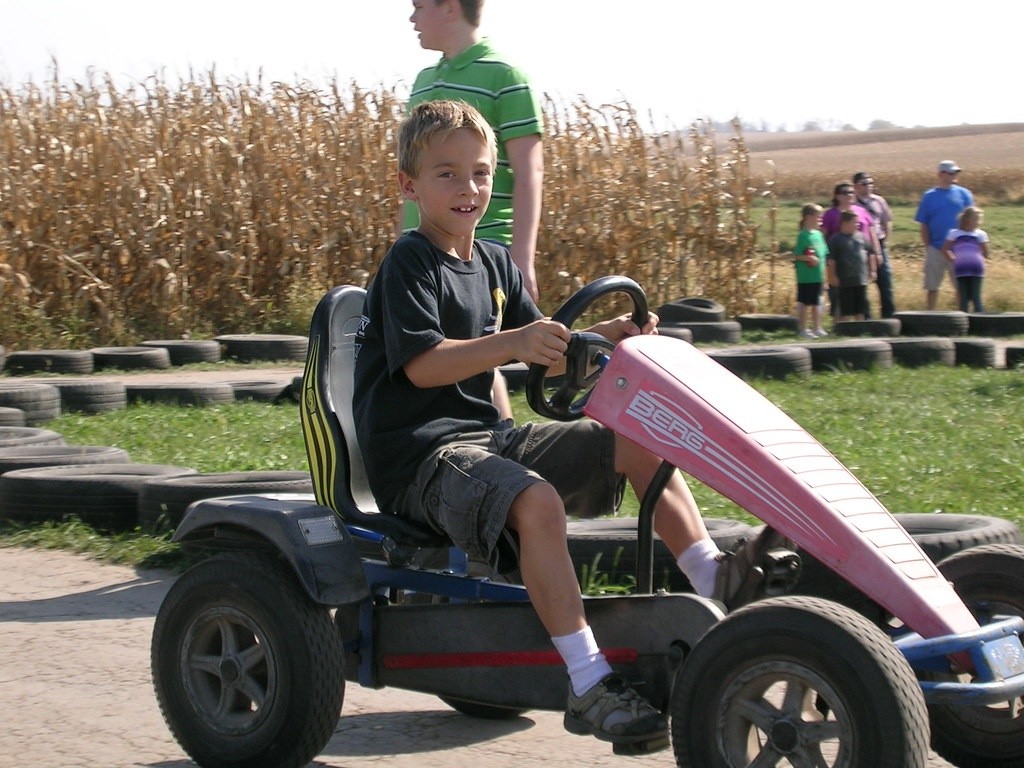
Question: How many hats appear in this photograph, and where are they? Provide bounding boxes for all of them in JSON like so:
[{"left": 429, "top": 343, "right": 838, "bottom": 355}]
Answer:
[{"left": 938, "top": 160, "right": 960, "bottom": 173}]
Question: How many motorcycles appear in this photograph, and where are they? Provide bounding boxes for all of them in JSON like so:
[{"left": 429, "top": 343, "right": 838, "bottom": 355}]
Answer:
[{"left": 145, "top": 270, "right": 1024, "bottom": 768}]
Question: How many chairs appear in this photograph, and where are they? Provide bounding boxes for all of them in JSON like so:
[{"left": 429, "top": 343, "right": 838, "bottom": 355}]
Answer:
[{"left": 301, "top": 284, "right": 455, "bottom": 549}]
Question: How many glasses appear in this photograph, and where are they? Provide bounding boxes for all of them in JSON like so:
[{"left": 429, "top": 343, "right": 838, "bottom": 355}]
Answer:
[
  {"left": 841, "top": 191, "right": 854, "bottom": 195},
  {"left": 862, "top": 182, "right": 873, "bottom": 185}
]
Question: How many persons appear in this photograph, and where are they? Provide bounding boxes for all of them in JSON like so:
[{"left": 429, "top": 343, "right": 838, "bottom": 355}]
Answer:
[
  {"left": 790, "top": 160, "right": 991, "bottom": 341},
  {"left": 351, "top": 97, "right": 804, "bottom": 742},
  {"left": 397, "top": 0, "right": 545, "bottom": 429}
]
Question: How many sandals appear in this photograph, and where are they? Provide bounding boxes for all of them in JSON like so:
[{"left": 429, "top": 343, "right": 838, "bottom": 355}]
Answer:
[
  {"left": 706, "top": 525, "right": 799, "bottom": 612},
  {"left": 563, "top": 671, "right": 669, "bottom": 745}
]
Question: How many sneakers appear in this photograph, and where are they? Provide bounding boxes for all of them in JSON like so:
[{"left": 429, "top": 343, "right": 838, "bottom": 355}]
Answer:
[
  {"left": 800, "top": 330, "right": 820, "bottom": 338},
  {"left": 813, "top": 329, "right": 829, "bottom": 337}
]
